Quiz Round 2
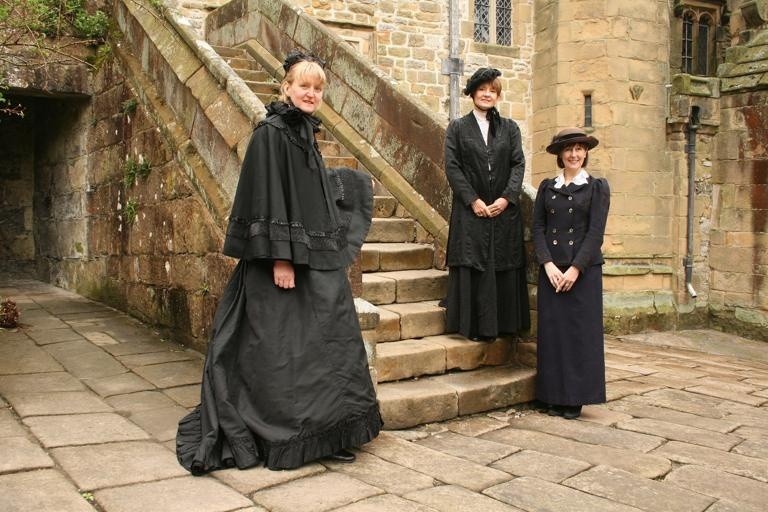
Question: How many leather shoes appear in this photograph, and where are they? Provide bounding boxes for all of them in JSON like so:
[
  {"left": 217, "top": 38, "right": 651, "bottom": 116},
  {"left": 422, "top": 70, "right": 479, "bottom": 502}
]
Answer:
[
  {"left": 324, "top": 450, "right": 356, "bottom": 463},
  {"left": 548, "top": 404, "right": 583, "bottom": 420}
]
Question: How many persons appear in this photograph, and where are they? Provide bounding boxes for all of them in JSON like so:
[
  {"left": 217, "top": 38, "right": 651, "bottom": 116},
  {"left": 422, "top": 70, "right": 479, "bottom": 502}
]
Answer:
[
  {"left": 536, "top": 128, "right": 610, "bottom": 419},
  {"left": 174, "top": 51, "right": 384, "bottom": 476},
  {"left": 443, "top": 65, "right": 531, "bottom": 339}
]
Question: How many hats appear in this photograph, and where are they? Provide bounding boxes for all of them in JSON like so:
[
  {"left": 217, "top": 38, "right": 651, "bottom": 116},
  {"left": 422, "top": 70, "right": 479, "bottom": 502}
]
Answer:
[
  {"left": 464, "top": 68, "right": 499, "bottom": 95},
  {"left": 546, "top": 126, "right": 599, "bottom": 155}
]
